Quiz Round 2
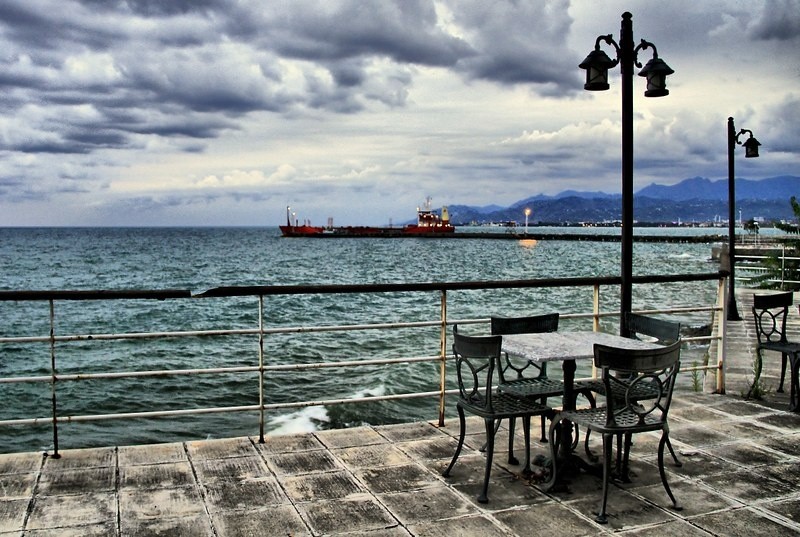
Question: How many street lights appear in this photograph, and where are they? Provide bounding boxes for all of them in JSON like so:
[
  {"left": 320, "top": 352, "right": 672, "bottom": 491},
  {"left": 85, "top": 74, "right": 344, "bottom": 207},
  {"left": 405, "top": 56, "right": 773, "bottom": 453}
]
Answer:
[
  {"left": 524, "top": 207, "right": 531, "bottom": 233},
  {"left": 725, "top": 116, "right": 761, "bottom": 322},
  {"left": 577, "top": 9, "right": 677, "bottom": 406}
]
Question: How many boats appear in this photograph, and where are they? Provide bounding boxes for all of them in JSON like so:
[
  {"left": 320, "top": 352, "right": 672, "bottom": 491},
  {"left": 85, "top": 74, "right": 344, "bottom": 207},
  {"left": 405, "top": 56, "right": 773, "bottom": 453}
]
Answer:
[{"left": 277, "top": 195, "right": 457, "bottom": 240}]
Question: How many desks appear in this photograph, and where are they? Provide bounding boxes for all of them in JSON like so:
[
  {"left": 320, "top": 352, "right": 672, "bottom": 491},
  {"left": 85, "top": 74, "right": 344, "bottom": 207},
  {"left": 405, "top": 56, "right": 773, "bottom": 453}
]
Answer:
[{"left": 470, "top": 331, "right": 669, "bottom": 479}]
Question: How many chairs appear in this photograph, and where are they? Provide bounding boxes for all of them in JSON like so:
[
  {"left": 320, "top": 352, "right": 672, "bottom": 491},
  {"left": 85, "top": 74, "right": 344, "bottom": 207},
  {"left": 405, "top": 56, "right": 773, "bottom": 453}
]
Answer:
[
  {"left": 743, "top": 289, "right": 800, "bottom": 411},
  {"left": 489, "top": 312, "right": 598, "bottom": 466},
  {"left": 442, "top": 323, "right": 554, "bottom": 506},
  {"left": 540, "top": 336, "right": 684, "bottom": 525},
  {"left": 575, "top": 312, "right": 685, "bottom": 470}
]
